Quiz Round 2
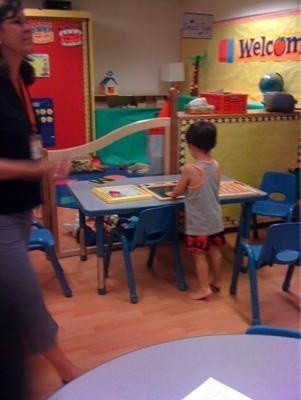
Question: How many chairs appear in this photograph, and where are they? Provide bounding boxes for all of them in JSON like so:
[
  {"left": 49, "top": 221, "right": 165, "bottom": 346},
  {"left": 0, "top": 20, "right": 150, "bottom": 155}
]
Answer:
[
  {"left": 229, "top": 170, "right": 301, "bottom": 326},
  {"left": 104, "top": 202, "right": 187, "bottom": 304},
  {"left": 26, "top": 220, "right": 74, "bottom": 297}
]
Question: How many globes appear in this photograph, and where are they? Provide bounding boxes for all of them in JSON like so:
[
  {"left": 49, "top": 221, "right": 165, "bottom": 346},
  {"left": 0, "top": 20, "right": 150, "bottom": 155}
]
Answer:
[{"left": 259, "top": 72, "right": 284, "bottom": 93}]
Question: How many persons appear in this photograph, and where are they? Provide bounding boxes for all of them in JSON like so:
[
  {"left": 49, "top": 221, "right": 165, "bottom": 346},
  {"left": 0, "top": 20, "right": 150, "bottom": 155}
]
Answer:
[
  {"left": 165, "top": 119, "right": 226, "bottom": 301},
  {"left": 0, "top": 0, "right": 88, "bottom": 395}
]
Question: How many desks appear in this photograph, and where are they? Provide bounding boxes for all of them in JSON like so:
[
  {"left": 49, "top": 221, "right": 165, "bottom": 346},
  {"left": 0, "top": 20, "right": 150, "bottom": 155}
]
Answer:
[
  {"left": 47, "top": 332, "right": 300, "bottom": 399},
  {"left": 65, "top": 172, "right": 268, "bottom": 296}
]
